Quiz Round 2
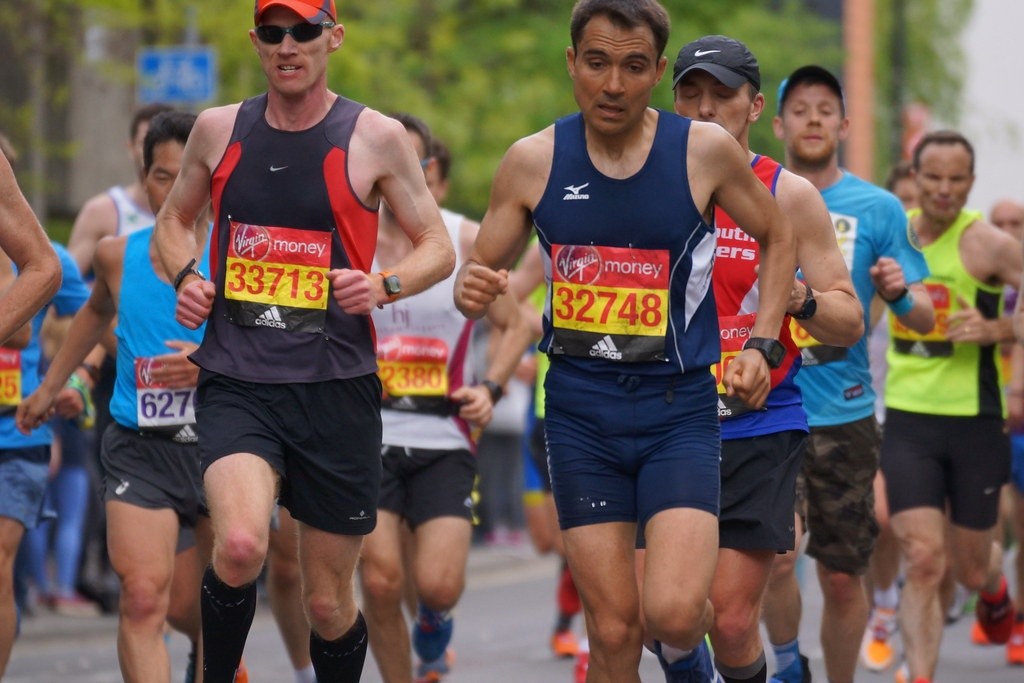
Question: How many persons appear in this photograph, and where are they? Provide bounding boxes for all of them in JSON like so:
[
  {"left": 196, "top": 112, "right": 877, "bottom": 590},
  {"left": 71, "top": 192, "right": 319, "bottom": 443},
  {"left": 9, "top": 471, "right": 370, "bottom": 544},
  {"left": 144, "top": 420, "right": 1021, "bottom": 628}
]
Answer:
[{"left": 0, "top": 0, "right": 1024, "bottom": 683}]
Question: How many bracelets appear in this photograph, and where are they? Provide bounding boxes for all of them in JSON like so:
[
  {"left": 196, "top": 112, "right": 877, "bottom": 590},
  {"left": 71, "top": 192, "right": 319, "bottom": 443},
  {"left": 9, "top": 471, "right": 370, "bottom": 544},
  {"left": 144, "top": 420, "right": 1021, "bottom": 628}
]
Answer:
[
  {"left": 876, "top": 286, "right": 908, "bottom": 305},
  {"left": 886, "top": 292, "right": 914, "bottom": 315}
]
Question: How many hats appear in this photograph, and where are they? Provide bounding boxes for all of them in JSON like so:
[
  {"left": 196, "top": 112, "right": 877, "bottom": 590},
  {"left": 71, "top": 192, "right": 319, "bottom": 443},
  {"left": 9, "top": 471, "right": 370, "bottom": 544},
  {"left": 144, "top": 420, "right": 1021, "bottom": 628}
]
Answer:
[
  {"left": 672, "top": 34, "right": 761, "bottom": 91},
  {"left": 253, "top": 0, "right": 337, "bottom": 26},
  {"left": 777, "top": 65, "right": 845, "bottom": 118}
]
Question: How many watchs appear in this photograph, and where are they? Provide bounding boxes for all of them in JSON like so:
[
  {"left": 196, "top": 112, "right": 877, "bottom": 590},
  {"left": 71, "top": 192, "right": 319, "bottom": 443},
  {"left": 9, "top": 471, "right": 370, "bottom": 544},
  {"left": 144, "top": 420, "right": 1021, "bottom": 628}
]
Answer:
[
  {"left": 742, "top": 337, "right": 787, "bottom": 368},
  {"left": 481, "top": 378, "right": 504, "bottom": 406},
  {"left": 376, "top": 270, "right": 401, "bottom": 309},
  {"left": 78, "top": 361, "right": 100, "bottom": 385},
  {"left": 785, "top": 286, "right": 817, "bottom": 320},
  {"left": 174, "top": 258, "right": 206, "bottom": 292}
]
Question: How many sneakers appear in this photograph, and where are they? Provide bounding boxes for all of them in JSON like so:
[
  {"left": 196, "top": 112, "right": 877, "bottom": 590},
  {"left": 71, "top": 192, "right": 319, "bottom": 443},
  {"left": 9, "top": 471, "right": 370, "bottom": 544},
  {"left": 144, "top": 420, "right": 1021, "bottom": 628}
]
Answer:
[{"left": 412, "top": 601, "right": 453, "bottom": 662}]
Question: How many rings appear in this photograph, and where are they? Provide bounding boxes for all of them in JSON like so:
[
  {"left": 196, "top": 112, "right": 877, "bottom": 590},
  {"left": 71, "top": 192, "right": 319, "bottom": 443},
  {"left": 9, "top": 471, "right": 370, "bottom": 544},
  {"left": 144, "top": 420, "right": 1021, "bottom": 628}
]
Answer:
[{"left": 964, "top": 327, "right": 970, "bottom": 332}]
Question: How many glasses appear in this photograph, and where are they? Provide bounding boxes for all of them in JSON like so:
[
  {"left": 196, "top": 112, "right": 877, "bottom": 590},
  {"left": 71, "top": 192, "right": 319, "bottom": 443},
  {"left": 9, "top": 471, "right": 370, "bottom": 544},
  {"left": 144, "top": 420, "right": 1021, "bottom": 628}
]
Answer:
[{"left": 256, "top": 21, "right": 335, "bottom": 45}]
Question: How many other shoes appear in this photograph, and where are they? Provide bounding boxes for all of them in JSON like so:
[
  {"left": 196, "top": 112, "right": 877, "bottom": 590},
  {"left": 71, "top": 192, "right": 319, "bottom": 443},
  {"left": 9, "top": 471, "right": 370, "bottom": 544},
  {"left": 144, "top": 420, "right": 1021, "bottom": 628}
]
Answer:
[
  {"left": 52, "top": 595, "right": 99, "bottom": 619},
  {"left": 549, "top": 630, "right": 580, "bottom": 658},
  {"left": 976, "top": 577, "right": 1015, "bottom": 644},
  {"left": 860, "top": 608, "right": 901, "bottom": 673},
  {"left": 656, "top": 638, "right": 721, "bottom": 683},
  {"left": 971, "top": 603, "right": 1024, "bottom": 666},
  {"left": 417, "top": 650, "right": 455, "bottom": 683}
]
